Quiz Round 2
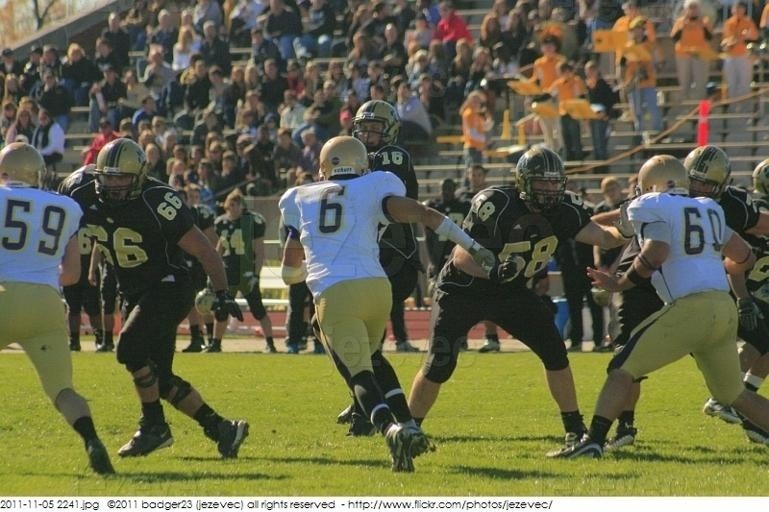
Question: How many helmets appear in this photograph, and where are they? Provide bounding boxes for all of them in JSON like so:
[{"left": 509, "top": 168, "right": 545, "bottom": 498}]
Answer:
[
  {"left": 0, "top": 142, "right": 47, "bottom": 187},
  {"left": 751, "top": 159, "right": 769, "bottom": 197},
  {"left": 93, "top": 138, "right": 149, "bottom": 202},
  {"left": 514, "top": 147, "right": 567, "bottom": 202},
  {"left": 682, "top": 144, "right": 731, "bottom": 197},
  {"left": 195, "top": 288, "right": 220, "bottom": 315},
  {"left": 628, "top": 14, "right": 647, "bottom": 33},
  {"left": 351, "top": 99, "right": 400, "bottom": 147},
  {"left": 318, "top": 135, "right": 370, "bottom": 181},
  {"left": 636, "top": 154, "right": 691, "bottom": 200}
]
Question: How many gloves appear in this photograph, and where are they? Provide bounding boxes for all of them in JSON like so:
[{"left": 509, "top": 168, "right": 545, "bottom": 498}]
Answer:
[
  {"left": 467, "top": 240, "right": 496, "bottom": 274},
  {"left": 610, "top": 199, "right": 639, "bottom": 239},
  {"left": 487, "top": 256, "right": 519, "bottom": 280},
  {"left": 248, "top": 275, "right": 260, "bottom": 293},
  {"left": 735, "top": 295, "right": 765, "bottom": 332},
  {"left": 214, "top": 287, "right": 245, "bottom": 322}
]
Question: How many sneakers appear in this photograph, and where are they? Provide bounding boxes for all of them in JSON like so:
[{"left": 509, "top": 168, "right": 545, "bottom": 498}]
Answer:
[
  {"left": 86, "top": 438, "right": 114, "bottom": 477},
  {"left": 335, "top": 402, "right": 382, "bottom": 438},
  {"left": 384, "top": 420, "right": 430, "bottom": 474},
  {"left": 182, "top": 341, "right": 222, "bottom": 354},
  {"left": 94, "top": 335, "right": 115, "bottom": 353},
  {"left": 218, "top": 418, "right": 251, "bottom": 459},
  {"left": 69, "top": 340, "right": 81, "bottom": 351},
  {"left": 478, "top": 337, "right": 500, "bottom": 353},
  {"left": 565, "top": 342, "right": 583, "bottom": 352},
  {"left": 395, "top": 340, "right": 419, "bottom": 352},
  {"left": 264, "top": 343, "right": 278, "bottom": 353},
  {"left": 702, "top": 395, "right": 743, "bottom": 425},
  {"left": 592, "top": 341, "right": 615, "bottom": 353},
  {"left": 117, "top": 421, "right": 174, "bottom": 459},
  {"left": 542, "top": 422, "right": 636, "bottom": 460},
  {"left": 287, "top": 342, "right": 299, "bottom": 354}
]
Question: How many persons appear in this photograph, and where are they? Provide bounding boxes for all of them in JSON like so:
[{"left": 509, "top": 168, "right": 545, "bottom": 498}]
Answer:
[
  {"left": 1, "top": 143, "right": 117, "bottom": 477},
  {"left": 0, "top": 0, "right": 768, "bottom": 355},
  {"left": 278, "top": 133, "right": 499, "bottom": 474},
  {"left": 546, "top": 153, "right": 768, "bottom": 460},
  {"left": 72, "top": 137, "right": 249, "bottom": 458},
  {"left": 607, "top": 143, "right": 767, "bottom": 447},
  {"left": 412, "top": 147, "right": 635, "bottom": 444},
  {"left": 704, "top": 155, "right": 768, "bottom": 425},
  {"left": 335, "top": 99, "right": 426, "bottom": 437}
]
